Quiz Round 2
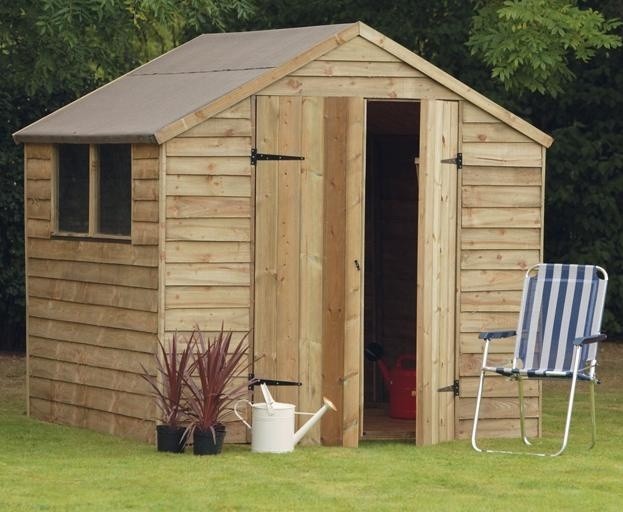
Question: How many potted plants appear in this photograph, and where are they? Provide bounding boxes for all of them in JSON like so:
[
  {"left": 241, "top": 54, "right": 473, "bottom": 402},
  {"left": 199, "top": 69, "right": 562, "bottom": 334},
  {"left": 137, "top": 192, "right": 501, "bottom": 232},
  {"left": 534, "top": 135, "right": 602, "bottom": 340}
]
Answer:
[
  {"left": 178, "top": 324, "right": 265, "bottom": 455},
  {"left": 139, "top": 332, "right": 218, "bottom": 453}
]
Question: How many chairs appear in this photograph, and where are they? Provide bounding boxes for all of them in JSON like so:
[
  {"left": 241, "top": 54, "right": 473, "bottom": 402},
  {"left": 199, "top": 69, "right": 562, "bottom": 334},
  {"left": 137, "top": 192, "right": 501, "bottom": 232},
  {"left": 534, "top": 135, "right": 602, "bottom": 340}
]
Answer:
[{"left": 471, "top": 262, "right": 608, "bottom": 457}]
